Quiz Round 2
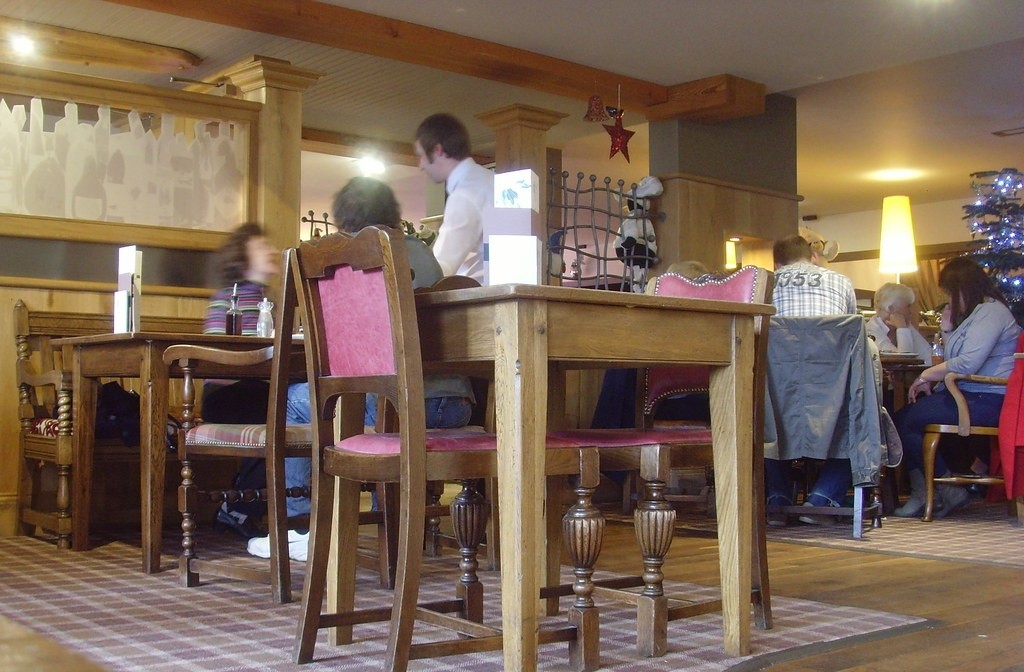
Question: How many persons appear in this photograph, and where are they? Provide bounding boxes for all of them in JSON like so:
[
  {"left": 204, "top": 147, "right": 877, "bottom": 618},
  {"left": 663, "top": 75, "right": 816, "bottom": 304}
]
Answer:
[
  {"left": 247, "top": 174, "right": 476, "bottom": 562},
  {"left": 864, "top": 281, "right": 940, "bottom": 413},
  {"left": 412, "top": 113, "right": 493, "bottom": 542},
  {"left": 895, "top": 256, "right": 1023, "bottom": 516},
  {"left": 201, "top": 221, "right": 275, "bottom": 538},
  {"left": 590, "top": 260, "right": 711, "bottom": 483},
  {"left": 763, "top": 235, "right": 858, "bottom": 526}
]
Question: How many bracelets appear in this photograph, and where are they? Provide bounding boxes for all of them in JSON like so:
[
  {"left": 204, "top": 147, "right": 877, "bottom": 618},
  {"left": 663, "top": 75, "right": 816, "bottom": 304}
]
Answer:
[
  {"left": 942, "top": 328, "right": 954, "bottom": 333},
  {"left": 918, "top": 376, "right": 926, "bottom": 382}
]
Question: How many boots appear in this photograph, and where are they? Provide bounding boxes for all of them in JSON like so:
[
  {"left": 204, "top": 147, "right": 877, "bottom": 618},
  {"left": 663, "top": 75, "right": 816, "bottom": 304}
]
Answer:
[
  {"left": 894, "top": 468, "right": 943, "bottom": 517},
  {"left": 932, "top": 470, "right": 971, "bottom": 517}
]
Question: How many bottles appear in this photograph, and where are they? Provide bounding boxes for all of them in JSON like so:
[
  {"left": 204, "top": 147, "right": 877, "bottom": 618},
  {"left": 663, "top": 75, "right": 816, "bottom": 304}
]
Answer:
[
  {"left": 225, "top": 295, "right": 242, "bottom": 336},
  {"left": 932, "top": 333, "right": 944, "bottom": 365},
  {"left": 256, "top": 301, "right": 274, "bottom": 338}
]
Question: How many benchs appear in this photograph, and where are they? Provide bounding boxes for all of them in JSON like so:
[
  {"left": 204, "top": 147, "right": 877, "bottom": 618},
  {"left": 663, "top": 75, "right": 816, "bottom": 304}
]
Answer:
[{"left": 12, "top": 300, "right": 305, "bottom": 550}]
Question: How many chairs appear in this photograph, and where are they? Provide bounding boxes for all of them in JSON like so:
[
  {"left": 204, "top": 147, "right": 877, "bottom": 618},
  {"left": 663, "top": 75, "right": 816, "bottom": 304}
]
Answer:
[
  {"left": 161, "top": 247, "right": 405, "bottom": 607},
  {"left": 547, "top": 261, "right": 1024, "bottom": 656},
  {"left": 285, "top": 222, "right": 601, "bottom": 672}
]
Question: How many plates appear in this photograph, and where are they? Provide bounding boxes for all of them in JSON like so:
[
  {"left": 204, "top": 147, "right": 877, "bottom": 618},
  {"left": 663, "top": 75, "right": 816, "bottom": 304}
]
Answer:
[{"left": 880, "top": 352, "right": 920, "bottom": 358}]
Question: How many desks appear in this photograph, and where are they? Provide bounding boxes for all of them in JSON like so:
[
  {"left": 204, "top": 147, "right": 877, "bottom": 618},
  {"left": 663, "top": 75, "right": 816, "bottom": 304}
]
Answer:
[
  {"left": 326, "top": 282, "right": 776, "bottom": 672},
  {"left": 882, "top": 361, "right": 933, "bottom": 496},
  {"left": 49, "top": 330, "right": 313, "bottom": 575}
]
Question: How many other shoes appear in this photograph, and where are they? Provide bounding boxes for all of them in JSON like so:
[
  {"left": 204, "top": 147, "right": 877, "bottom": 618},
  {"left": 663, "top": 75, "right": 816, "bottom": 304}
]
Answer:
[
  {"left": 212, "top": 500, "right": 266, "bottom": 539},
  {"left": 799, "top": 501, "right": 836, "bottom": 525},
  {"left": 767, "top": 513, "right": 788, "bottom": 526}
]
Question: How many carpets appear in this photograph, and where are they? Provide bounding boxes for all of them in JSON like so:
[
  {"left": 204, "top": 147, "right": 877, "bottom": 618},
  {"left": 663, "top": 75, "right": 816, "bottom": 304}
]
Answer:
[
  {"left": 560, "top": 491, "right": 1024, "bottom": 571},
  {"left": 0, "top": 533, "right": 947, "bottom": 672}
]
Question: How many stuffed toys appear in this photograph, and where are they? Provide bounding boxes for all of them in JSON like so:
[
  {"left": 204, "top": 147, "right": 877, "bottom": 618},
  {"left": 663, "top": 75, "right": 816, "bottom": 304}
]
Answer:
[{"left": 613, "top": 175, "right": 664, "bottom": 293}]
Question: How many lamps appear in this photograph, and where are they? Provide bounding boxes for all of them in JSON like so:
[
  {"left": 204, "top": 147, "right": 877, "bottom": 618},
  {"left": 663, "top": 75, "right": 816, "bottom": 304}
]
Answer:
[{"left": 878, "top": 196, "right": 918, "bottom": 285}]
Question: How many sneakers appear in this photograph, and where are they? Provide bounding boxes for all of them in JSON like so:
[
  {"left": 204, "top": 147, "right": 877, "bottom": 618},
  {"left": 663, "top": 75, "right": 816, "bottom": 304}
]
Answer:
[{"left": 247, "top": 528, "right": 310, "bottom": 561}]
